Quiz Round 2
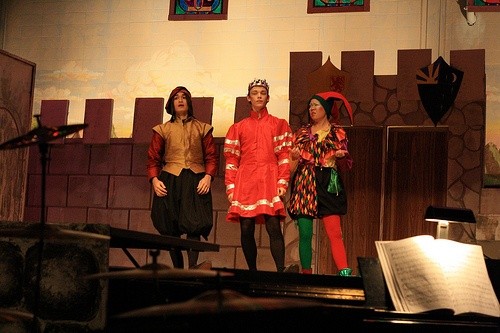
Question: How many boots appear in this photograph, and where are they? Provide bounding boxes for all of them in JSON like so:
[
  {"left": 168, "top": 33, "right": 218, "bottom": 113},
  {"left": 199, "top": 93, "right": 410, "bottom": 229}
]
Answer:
[
  {"left": 338, "top": 268, "right": 352, "bottom": 277},
  {"left": 302, "top": 268, "right": 313, "bottom": 275}
]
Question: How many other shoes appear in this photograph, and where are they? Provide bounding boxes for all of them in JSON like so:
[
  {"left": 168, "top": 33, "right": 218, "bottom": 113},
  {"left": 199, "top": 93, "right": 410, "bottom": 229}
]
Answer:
[{"left": 282, "top": 263, "right": 300, "bottom": 273}]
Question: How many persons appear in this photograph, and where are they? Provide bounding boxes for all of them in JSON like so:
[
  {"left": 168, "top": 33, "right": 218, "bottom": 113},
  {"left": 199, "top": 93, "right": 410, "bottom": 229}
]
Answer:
[
  {"left": 224, "top": 79, "right": 293, "bottom": 273},
  {"left": 288, "top": 93, "right": 352, "bottom": 277},
  {"left": 147, "top": 87, "right": 218, "bottom": 268}
]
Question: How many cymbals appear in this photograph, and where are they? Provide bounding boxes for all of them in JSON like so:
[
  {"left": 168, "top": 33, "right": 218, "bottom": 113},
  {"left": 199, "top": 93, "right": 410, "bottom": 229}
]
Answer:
[
  {"left": 112, "top": 289, "right": 328, "bottom": 319},
  {"left": 78, "top": 268, "right": 234, "bottom": 280},
  {"left": 0, "top": 121, "right": 90, "bottom": 149}
]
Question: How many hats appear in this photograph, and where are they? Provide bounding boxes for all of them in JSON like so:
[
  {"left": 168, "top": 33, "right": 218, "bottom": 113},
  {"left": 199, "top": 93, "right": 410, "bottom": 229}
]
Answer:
[{"left": 248, "top": 79, "right": 270, "bottom": 92}]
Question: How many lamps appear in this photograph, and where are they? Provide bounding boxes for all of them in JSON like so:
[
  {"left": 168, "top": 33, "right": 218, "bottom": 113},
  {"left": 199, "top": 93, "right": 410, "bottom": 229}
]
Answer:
[{"left": 425, "top": 203, "right": 477, "bottom": 239}]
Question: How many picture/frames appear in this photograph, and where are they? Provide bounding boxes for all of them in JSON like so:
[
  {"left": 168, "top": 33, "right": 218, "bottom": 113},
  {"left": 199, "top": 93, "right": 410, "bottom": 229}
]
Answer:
[
  {"left": 468, "top": 0, "right": 500, "bottom": 12},
  {"left": 168, "top": 0, "right": 229, "bottom": 21},
  {"left": 307, "top": 0, "right": 370, "bottom": 13}
]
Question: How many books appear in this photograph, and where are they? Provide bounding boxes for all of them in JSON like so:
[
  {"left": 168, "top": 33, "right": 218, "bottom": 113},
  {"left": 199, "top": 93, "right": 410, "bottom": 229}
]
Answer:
[{"left": 375, "top": 235, "right": 500, "bottom": 318}]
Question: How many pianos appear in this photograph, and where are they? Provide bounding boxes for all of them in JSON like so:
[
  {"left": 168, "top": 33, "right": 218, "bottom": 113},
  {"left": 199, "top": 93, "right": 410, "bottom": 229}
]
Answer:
[{"left": 209, "top": 263, "right": 500, "bottom": 333}]
